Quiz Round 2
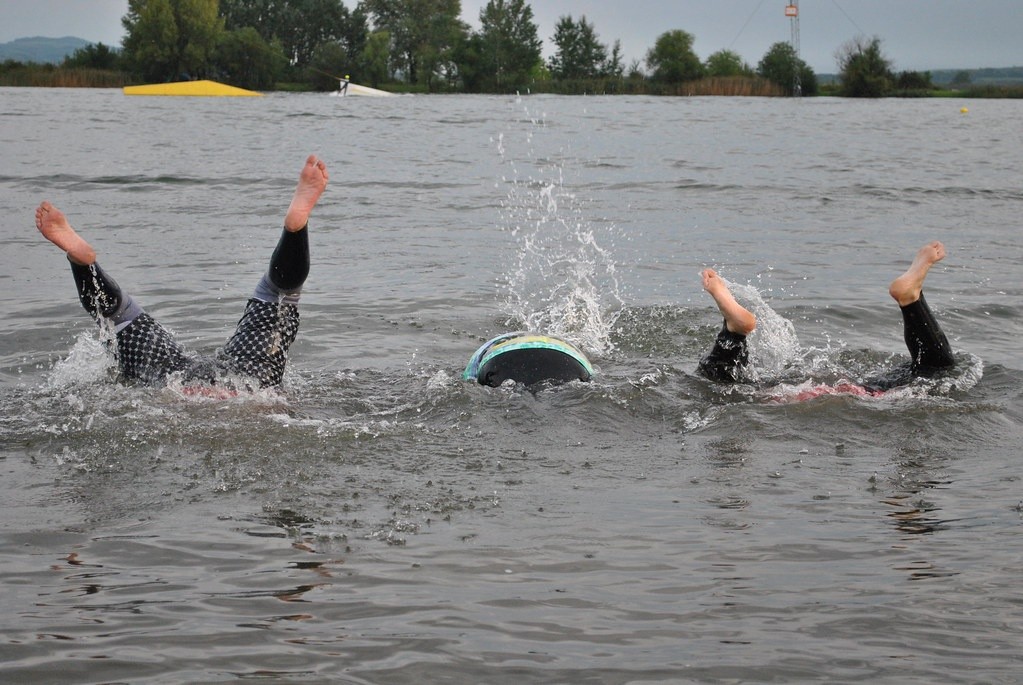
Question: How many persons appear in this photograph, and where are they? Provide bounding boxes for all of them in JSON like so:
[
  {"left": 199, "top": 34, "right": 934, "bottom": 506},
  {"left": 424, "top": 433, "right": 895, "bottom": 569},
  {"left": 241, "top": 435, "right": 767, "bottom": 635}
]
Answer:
[
  {"left": 465, "top": 329, "right": 593, "bottom": 384},
  {"left": 36, "top": 155, "right": 329, "bottom": 391},
  {"left": 696, "top": 239, "right": 953, "bottom": 382},
  {"left": 338, "top": 75, "right": 349, "bottom": 96}
]
